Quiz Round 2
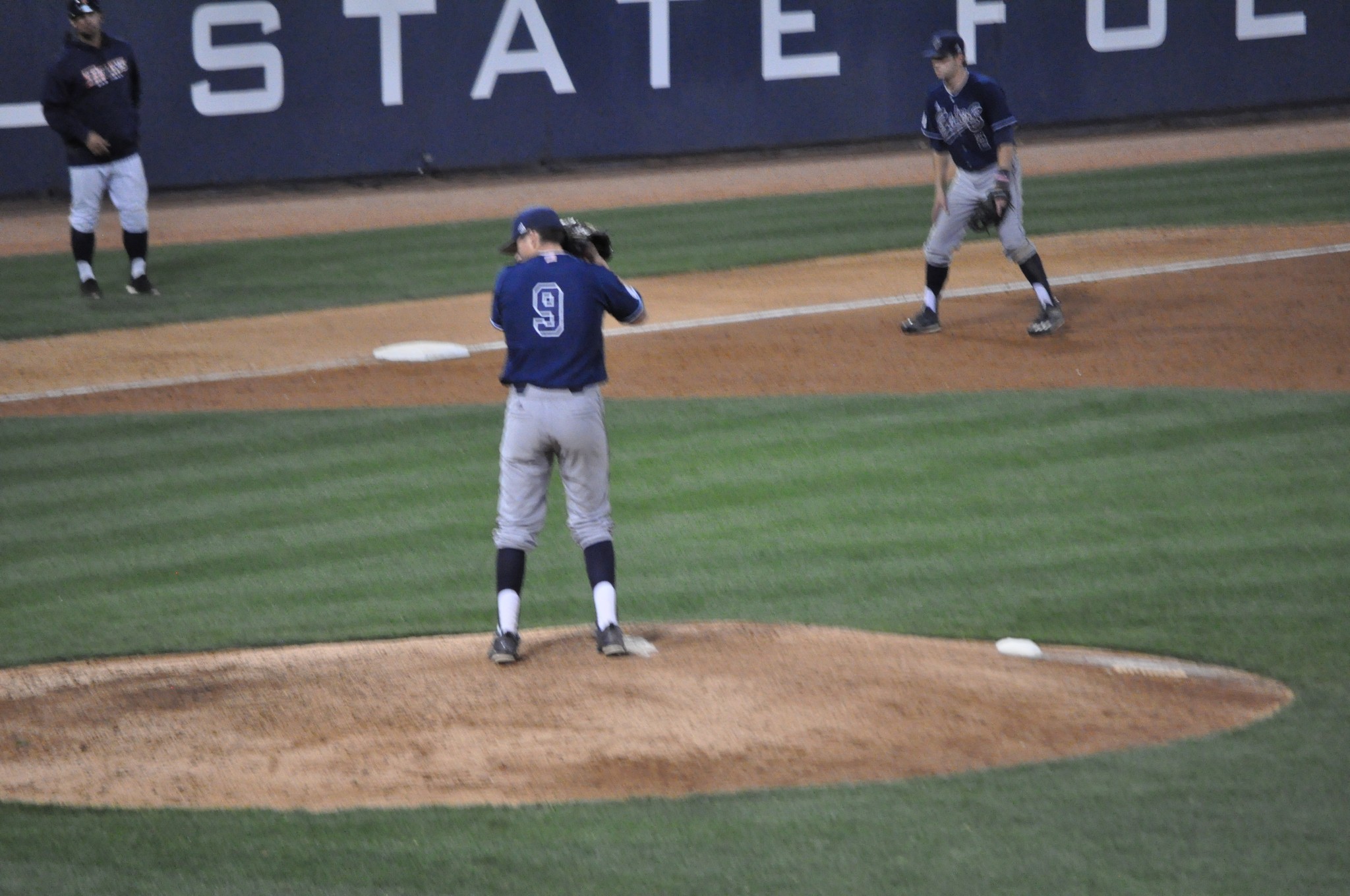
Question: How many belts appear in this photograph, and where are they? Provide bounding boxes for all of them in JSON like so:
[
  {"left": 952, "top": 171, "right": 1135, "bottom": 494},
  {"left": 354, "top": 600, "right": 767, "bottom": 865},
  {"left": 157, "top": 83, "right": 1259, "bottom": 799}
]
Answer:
[{"left": 516, "top": 383, "right": 585, "bottom": 393}]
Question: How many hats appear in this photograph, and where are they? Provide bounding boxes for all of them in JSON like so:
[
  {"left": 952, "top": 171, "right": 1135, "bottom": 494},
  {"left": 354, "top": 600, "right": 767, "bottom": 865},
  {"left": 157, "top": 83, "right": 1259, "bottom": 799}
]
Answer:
[
  {"left": 922, "top": 32, "right": 966, "bottom": 57},
  {"left": 500, "top": 208, "right": 567, "bottom": 252},
  {"left": 70, "top": 0, "right": 101, "bottom": 18}
]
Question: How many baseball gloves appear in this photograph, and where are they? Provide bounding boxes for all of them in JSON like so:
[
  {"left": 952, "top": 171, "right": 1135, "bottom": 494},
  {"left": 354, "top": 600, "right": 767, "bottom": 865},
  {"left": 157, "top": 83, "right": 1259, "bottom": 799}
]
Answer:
[
  {"left": 557, "top": 215, "right": 614, "bottom": 264},
  {"left": 967, "top": 186, "right": 1014, "bottom": 233}
]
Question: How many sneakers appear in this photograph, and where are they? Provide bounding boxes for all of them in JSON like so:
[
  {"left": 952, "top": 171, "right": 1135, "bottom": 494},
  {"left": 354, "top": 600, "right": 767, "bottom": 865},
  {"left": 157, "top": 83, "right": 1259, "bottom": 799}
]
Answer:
[
  {"left": 597, "top": 624, "right": 627, "bottom": 656},
  {"left": 486, "top": 632, "right": 518, "bottom": 662},
  {"left": 126, "top": 274, "right": 160, "bottom": 296},
  {"left": 1028, "top": 308, "right": 1063, "bottom": 336},
  {"left": 902, "top": 309, "right": 940, "bottom": 333},
  {"left": 79, "top": 278, "right": 102, "bottom": 297}
]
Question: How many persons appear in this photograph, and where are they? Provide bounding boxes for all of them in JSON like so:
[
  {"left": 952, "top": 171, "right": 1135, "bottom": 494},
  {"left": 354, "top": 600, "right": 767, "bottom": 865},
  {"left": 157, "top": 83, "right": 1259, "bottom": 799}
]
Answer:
[
  {"left": 896, "top": 33, "right": 1069, "bottom": 338},
  {"left": 41, "top": 0, "right": 163, "bottom": 302},
  {"left": 487, "top": 209, "right": 648, "bottom": 664}
]
